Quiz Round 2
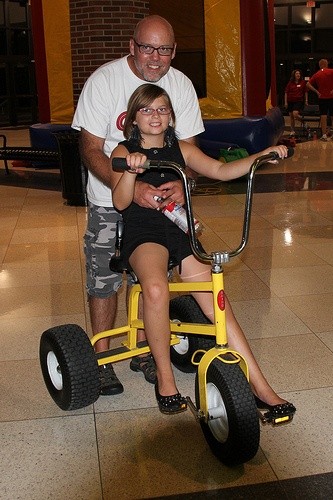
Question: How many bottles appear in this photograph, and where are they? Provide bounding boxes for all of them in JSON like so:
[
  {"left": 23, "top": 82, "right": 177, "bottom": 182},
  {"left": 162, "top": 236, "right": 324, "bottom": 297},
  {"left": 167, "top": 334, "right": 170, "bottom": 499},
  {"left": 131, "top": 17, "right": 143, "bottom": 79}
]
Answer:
[{"left": 154, "top": 195, "right": 205, "bottom": 239}]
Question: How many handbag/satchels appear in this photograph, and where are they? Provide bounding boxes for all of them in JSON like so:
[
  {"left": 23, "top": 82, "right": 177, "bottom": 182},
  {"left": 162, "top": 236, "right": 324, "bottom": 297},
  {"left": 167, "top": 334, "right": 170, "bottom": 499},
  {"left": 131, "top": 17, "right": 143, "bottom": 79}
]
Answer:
[{"left": 221, "top": 144, "right": 249, "bottom": 162}]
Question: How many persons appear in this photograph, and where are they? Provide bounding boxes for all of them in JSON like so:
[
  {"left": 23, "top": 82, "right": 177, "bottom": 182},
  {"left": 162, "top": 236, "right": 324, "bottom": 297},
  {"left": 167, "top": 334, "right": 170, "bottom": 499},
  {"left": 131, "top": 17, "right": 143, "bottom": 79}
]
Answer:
[
  {"left": 109, "top": 84, "right": 296, "bottom": 417},
  {"left": 70, "top": 14, "right": 206, "bottom": 397},
  {"left": 306, "top": 59, "right": 333, "bottom": 142},
  {"left": 284, "top": 69, "right": 308, "bottom": 136}
]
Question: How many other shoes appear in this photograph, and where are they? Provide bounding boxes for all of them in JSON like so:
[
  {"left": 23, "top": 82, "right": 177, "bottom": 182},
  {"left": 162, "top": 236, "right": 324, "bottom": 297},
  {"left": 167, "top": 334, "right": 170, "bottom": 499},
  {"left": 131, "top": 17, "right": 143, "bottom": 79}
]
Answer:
[
  {"left": 290, "top": 131, "right": 295, "bottom": 136},
  {"left": 155, "top": 383, "right": 188, "bottom": 415},
  {"left": 320, "top": 135, "right": 327, "bottom": 142},
  {"left": 254, "top": 394, "right": 296, "bottom": 416}
]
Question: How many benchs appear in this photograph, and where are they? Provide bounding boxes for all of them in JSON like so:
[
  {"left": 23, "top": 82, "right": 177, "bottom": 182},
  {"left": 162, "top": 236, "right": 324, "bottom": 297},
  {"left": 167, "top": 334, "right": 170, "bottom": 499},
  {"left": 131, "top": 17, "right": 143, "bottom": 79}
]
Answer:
[{"left": 31, "top": 115, "right": 285, "bottom": 169}]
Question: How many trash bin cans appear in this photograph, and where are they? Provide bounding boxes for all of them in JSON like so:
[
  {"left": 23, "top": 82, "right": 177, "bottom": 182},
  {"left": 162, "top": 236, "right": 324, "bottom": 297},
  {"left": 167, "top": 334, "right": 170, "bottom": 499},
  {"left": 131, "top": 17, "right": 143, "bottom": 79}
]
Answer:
[{"left": 52, "top": 129, "right": 89, "bottom": 207}]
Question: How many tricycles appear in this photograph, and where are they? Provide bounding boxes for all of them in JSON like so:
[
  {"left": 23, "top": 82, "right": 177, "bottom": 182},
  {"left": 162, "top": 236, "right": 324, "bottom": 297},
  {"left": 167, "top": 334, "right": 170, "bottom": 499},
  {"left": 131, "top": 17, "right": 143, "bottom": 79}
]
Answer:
[{"left": 39, "top": 156, "right": 299, "bottom": 466}]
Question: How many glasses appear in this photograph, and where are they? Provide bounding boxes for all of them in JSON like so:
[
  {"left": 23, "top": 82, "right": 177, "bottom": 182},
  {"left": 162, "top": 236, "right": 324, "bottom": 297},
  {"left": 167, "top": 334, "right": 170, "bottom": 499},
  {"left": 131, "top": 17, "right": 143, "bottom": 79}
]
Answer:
[
  {"left": 133, "top": 38, "right": 174, "bottom": 56},
  {"left": 139, "top": 107, "right": 172, "bottom": 115}
]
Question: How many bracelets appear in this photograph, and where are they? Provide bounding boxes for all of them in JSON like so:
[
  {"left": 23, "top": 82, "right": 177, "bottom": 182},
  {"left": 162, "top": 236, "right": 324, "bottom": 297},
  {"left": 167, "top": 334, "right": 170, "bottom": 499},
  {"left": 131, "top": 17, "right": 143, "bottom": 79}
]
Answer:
[{"left": 127, "top": 170, "right": 137, "bottom": 174}]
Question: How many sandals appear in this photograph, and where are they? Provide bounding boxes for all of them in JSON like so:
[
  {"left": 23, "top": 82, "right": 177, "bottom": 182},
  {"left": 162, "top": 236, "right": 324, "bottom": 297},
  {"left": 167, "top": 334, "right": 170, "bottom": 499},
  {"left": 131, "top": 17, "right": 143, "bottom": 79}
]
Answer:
[
  {"left": 98, "top": 363, "right": 124, "bottom": 396},
  {"left": 130, "top": 354, "right": 158, "bottom": 384}
]
